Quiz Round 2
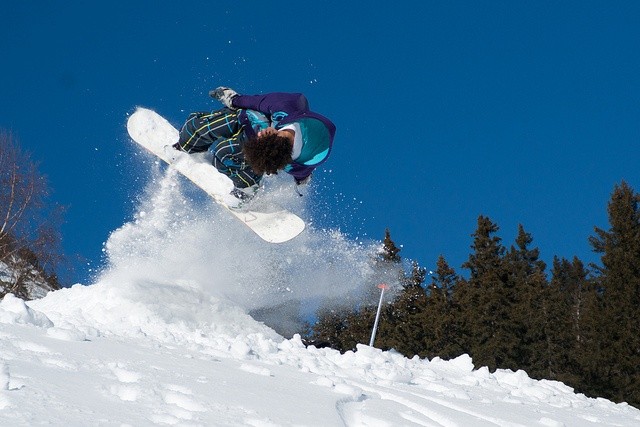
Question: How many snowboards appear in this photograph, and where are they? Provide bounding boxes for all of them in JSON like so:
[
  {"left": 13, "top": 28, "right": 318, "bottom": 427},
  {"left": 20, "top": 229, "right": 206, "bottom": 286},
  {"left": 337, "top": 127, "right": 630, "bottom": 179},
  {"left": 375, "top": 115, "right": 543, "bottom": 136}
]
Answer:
[{"left": 127, "top": 108, "right": 305, "bottom": 244}]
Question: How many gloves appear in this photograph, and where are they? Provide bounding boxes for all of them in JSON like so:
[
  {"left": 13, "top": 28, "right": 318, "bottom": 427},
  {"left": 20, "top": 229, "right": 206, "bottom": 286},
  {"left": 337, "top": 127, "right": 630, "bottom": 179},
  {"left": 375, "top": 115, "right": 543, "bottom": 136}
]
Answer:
[
  {"left": 209, "top": 85, "right": 240, "bottom": 111},
  {"left": 294, "top": 174, "right": 311, "bottom": 184}
]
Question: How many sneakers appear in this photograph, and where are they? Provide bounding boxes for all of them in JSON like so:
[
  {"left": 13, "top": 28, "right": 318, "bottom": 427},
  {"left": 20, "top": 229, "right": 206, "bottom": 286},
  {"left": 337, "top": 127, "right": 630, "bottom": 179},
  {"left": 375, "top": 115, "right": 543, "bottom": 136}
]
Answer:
[
  {"left": 165, "top": 142, "right": 185, "bottom": 160},
  {"left": 228, "top": 184, "right": 260, "bottom": 209}
]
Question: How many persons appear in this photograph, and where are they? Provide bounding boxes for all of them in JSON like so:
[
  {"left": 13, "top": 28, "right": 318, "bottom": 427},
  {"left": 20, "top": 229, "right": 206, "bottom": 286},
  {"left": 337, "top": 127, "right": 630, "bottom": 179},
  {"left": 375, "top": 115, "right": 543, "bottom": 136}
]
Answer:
[{"left": 166, "top": 85, "right": 337, "bottom": 213}]
